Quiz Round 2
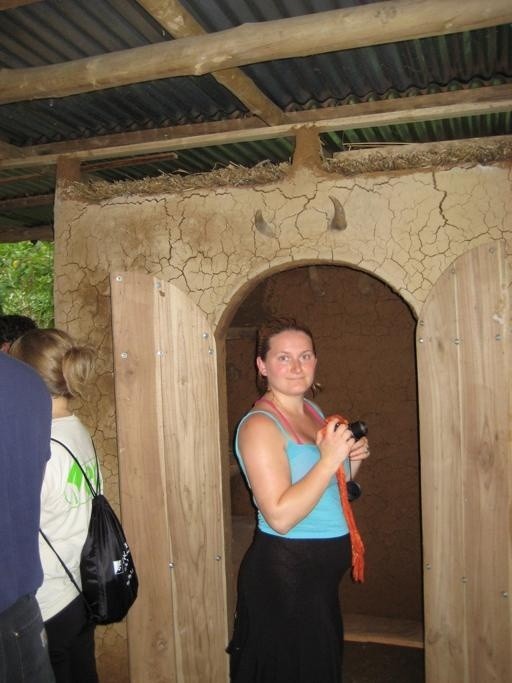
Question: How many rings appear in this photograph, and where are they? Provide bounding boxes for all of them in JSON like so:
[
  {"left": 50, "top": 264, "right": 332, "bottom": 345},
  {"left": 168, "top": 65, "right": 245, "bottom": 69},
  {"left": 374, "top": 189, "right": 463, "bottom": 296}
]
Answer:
[{"left": 364, "top": 447, "right": 369, "bottom": 453}]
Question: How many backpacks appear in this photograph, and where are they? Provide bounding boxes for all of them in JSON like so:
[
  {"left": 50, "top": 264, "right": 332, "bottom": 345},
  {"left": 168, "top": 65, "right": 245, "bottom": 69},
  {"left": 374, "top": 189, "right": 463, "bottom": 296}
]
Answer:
[{"left": 36, "top": 428, "right": 140, "bottom": 625}]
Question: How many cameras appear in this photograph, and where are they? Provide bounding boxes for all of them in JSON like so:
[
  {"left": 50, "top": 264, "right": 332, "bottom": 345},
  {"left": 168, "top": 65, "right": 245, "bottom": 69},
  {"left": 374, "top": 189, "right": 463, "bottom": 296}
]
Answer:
[{"left": 332, "top": 421, "right": 367, "bottom": 441}]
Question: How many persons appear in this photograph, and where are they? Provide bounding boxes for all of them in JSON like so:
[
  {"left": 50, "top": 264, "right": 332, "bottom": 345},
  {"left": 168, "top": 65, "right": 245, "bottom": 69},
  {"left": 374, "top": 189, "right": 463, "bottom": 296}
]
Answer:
[
  {"left": 9, "top": 325, "right": 102, "bottom": 682},
  {"left": 1, "top": 341, "right": 59, "bottom": 683},
  {"left": 0, "top": 313, "right": 41, "bottom": 355},
  {"left": 221, "top": 313, "right": 373, "bottom": 682}
]
